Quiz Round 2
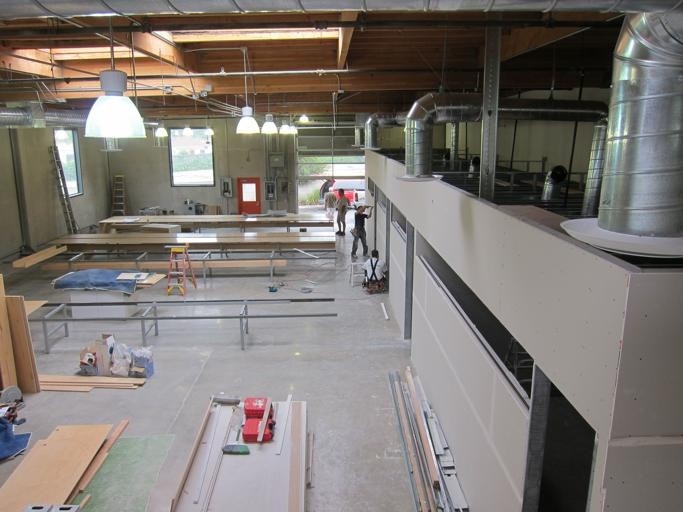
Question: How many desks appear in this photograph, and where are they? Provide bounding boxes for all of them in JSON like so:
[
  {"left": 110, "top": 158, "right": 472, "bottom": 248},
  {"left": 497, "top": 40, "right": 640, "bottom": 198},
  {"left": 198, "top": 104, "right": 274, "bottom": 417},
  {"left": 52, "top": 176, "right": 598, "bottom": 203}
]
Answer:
[
  {"left": 41, "top": 230, "right": 333, "bottom": 283},
  {"left": 99, "top": 215, "right": 335, "bottom": 234},
  {"left": 51, "top": 270, "right": 167, "bottom": 318}
]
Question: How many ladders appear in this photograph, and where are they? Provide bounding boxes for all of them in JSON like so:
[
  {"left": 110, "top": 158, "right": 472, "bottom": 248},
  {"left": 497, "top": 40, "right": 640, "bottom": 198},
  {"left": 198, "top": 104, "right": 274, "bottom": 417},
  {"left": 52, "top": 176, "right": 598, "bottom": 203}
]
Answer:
[
  {"left": 167, "top": 246, "right": 196, "bottom": 296},
  {"left": 48, "top": 145, "right": 77, "bottom": 234},
  {"left": 111, "top": 175, "right": 128, "bottom": 216}
]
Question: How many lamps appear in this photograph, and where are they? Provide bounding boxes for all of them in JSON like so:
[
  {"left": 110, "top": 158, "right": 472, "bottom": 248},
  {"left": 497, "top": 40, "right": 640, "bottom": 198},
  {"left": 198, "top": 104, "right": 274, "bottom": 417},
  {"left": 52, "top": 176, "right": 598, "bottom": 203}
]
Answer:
[
  {"left": 84, "top": 17, "right": 147, "bottom": 139},
  {"left": 234, "top": 49, "right": 298, "bottom": 135},
  {"left": 154, "top": 119, "right": 169, "bottom": 137}
]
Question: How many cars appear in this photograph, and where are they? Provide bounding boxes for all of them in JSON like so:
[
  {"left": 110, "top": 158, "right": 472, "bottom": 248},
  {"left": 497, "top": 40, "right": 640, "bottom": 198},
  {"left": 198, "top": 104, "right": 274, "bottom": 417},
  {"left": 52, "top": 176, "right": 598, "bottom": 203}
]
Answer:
[{"left": 329, "top": 180, "right": 365, "bottom": 209}]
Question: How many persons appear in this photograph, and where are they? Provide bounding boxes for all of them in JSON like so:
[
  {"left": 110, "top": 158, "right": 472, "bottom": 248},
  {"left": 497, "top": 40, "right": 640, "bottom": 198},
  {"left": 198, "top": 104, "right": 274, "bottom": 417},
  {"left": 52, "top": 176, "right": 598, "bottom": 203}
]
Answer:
[
  {"left": 361, "top": 248, "right": 388, "bottom": 288},
  {"left": 350, "top": 205, "right": 374, "bottom": 259},
  {"left": 322, "top": 186, "right": 337, "bottom": 223},
  {"left": 335, "top": 189, "right": 350, "bottom": 236}
]
